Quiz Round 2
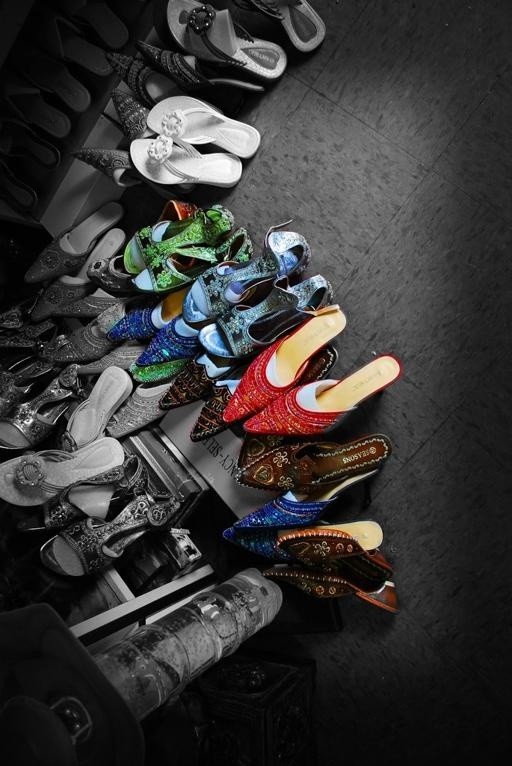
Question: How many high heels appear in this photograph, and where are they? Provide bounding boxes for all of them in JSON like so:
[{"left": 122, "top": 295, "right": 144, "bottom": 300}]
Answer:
[{"left": 234, "top": 306, "right": 402, "bottom": 615}]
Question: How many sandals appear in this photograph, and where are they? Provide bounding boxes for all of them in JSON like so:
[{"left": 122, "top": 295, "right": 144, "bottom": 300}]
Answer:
[
  {"left": 129, "top": 207, "right": 330, "bottom": 354},
  {"left": 0, "top": 214, "right": 182, "bottom": 572}
]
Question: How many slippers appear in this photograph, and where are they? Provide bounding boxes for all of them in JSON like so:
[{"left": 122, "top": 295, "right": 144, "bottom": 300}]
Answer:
[
  {"left": 9, "top": 3, "right": 132, "bottom": 208},
  {"left": 136, "top": 3, "right": 321, "bottom": 189}
]
